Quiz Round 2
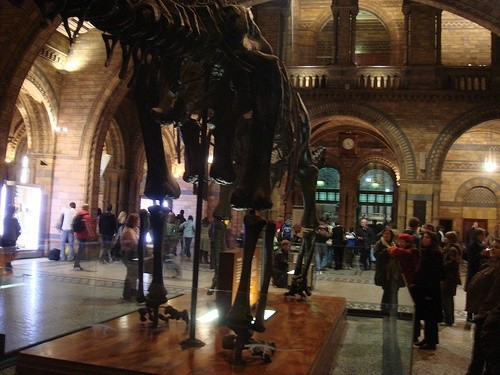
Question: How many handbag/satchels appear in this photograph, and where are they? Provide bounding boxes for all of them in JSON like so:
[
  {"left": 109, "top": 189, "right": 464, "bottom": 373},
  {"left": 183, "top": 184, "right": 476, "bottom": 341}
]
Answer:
[
  {"left": 48, "top": 249, "right": 61, "bottom": 261},
  {"left": 343, "top": 228, "right": 347, "bottom": 241},
  {"left": 111, "top": 236, "right": 121, "bottom": 261}
]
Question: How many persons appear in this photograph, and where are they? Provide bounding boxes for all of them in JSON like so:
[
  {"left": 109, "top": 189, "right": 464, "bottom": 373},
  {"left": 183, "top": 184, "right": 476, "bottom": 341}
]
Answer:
[
  {"left": 56, "top": 201, "right": 76, "bottom": 261},
  {"left": 315, "top": 219, "right": 372, "bottom": 271},
  {"left": 2, "top": 203, "right": 21, "bottom": 269},
  {"left": 119, "top": 213, "right": 140, "bottom": 301},
  {"left": 174, "top": 209, "right": 209, "bottom": 264},
  {"left": 271, "top": 240, "right": 291, "bottom": 288},
  {"left": 460, "top": 221, "right": 500, "bottom": 375},
  {"left": 99, "top": 204, "right": 117, "bottom": 262},
  {"left": 70, "top": 203, "right": 97, "bottom": 270},
  {"left": 373, "top": 217, "right": 463, "bottom": 350}
]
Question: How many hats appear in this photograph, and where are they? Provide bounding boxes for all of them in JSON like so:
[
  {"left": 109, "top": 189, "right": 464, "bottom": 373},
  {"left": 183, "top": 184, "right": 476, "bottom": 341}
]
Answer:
[{"left": 397, "top": 234, "right": 414, "bottom": 244}]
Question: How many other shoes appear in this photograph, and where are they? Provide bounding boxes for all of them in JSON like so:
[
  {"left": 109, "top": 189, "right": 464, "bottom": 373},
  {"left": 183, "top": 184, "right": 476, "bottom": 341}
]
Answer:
[
  {"left": 73, "top": 266, "right": 83, "bottom": 270},
  {"left": 413, "top": 339, "right": 436, "bottom": 350}
]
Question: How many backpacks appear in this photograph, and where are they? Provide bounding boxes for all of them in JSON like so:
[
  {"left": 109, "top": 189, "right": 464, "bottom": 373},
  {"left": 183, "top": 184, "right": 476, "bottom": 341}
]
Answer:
[
  {"left": 282, "top": 224, "right": 291, "bottom": 239},
  {"left": 71, "top": 213, "right": 90, "bottom": 233}
]
